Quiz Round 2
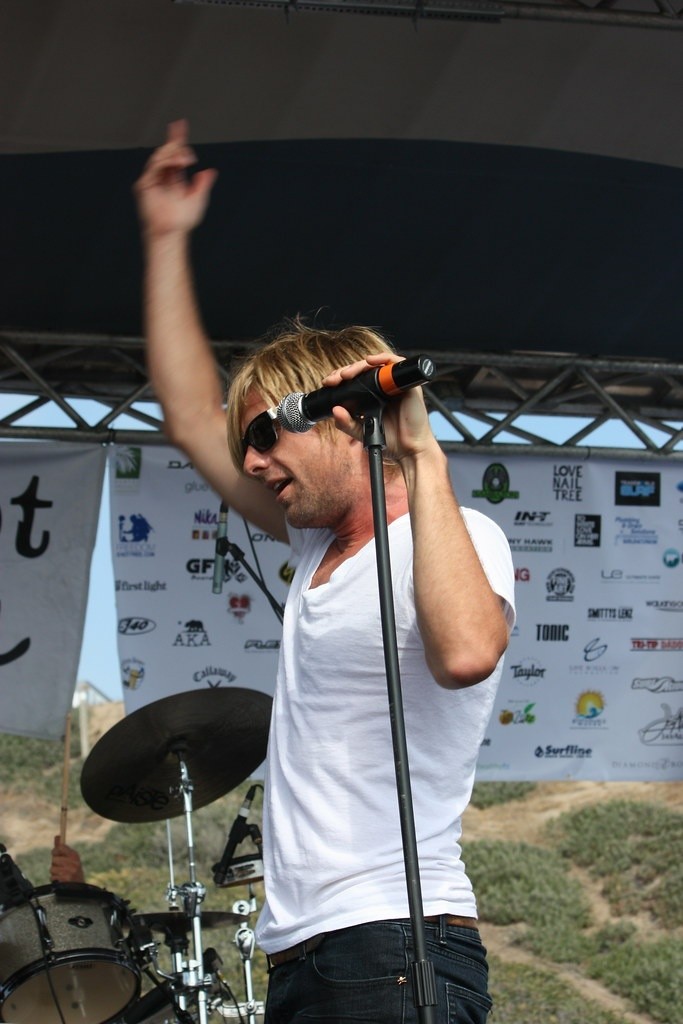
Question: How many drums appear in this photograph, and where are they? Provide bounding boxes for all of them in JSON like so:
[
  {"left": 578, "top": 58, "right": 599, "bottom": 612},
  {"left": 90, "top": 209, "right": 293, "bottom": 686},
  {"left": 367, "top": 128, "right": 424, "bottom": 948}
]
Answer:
[
  {"left": 126, "top": 971, "right": 228, "bottom": 1024},
  {"left": 0, "top": 883, "right": 144, "bottom": 1024}
]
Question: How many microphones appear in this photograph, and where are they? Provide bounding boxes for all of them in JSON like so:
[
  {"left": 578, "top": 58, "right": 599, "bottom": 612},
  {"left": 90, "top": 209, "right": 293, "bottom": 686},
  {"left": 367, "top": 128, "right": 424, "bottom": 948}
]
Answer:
[
  {"left": 210, "top": 502, "right": 229, "bottom": 594},
  {"left": 277, "top": 353, "right": 436, "bottom": 435},
  {"left": 213, "top": 787, "right": 257, "bottom": 884},
  {"left": 205, "top": 947, "right": 223, "bottom": 972}
]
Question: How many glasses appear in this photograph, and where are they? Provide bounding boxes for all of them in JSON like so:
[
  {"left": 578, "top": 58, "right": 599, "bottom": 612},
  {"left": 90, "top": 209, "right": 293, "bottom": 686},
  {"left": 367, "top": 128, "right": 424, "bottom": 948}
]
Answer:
[{"left": 241, "top": 405, "right": 279, "bottom": 459}]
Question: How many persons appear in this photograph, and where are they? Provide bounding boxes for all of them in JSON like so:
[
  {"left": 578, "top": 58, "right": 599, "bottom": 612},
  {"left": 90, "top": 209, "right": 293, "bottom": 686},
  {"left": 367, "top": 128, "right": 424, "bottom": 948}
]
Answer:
[
  {"left": 133, "top": 122, "right": 517, "bottom": 1024},
  {"left": 49, "top": 835, "right": 86, "bottom": 884}
]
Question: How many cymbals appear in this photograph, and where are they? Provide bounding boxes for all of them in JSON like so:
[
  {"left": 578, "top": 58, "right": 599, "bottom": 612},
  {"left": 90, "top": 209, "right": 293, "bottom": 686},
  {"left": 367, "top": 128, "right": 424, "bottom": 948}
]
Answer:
[
  {"left": 131, "top": 910, "right": 250, "bottom": 933},
  {"left": 79, "top": 686, "right": 276, "bottom": 825}
]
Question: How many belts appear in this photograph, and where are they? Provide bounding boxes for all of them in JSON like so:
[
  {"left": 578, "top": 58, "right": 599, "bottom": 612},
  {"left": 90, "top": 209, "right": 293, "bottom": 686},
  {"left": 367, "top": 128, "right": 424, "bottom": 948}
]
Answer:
[{"left": 268, "top": 915, "right": 479, "bottom": 968}]
